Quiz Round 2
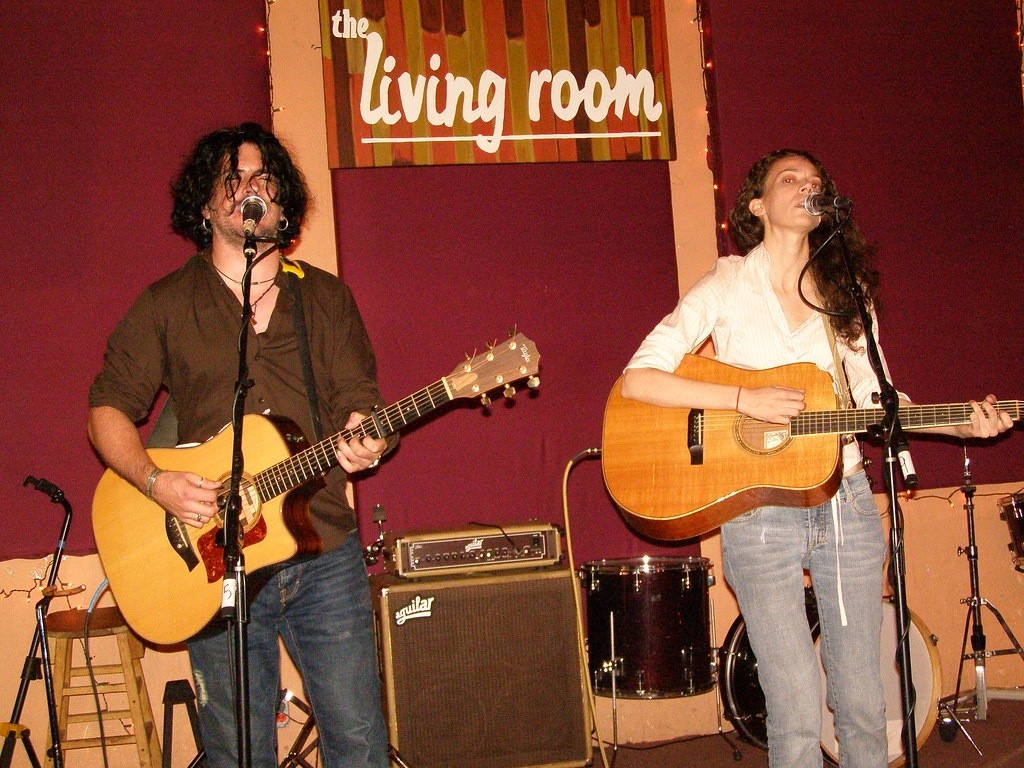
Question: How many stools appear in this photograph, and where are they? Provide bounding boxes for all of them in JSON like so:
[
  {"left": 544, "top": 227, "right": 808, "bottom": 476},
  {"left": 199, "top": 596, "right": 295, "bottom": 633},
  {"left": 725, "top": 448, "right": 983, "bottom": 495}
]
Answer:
[{"left": 45, "top": 606, "right": 163, "bottom": 768}]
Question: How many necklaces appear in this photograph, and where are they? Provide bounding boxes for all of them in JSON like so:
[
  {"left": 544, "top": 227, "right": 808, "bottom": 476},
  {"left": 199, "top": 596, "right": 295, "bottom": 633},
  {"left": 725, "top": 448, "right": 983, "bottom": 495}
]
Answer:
[
  {"left": 213, "top": 265, "right": 279, "bottom": 285},
  {"left": 250, "top": 283, "right": 274, "bottom": 325}
]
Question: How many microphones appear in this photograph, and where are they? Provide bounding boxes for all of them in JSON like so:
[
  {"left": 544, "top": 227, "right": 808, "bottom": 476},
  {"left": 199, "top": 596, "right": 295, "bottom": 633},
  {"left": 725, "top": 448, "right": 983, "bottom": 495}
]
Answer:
[
  {"left": 804, "top": 192, "right": 853, "bottom": 216},
  {"left": 241, "top": 196, "right": 268, "bottom": 236}
]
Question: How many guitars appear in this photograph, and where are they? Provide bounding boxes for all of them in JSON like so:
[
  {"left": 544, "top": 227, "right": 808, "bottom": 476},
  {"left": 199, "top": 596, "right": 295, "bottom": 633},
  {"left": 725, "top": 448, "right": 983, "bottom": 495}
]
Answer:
[
  {"left": 601, "top": 350, "right": 1024, "bottom": 545},
  {"left": 93, "top": 327, "right": 541, "bottom": 646}
]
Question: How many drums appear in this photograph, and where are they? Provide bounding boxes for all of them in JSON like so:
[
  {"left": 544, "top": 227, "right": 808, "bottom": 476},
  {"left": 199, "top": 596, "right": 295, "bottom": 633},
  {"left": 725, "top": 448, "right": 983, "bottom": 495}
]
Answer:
[
  {"left": 1000, "top": 493, "right": 1023, "bottom": 570},
  {"left": 584, "top": 557, "right": 711, "bottom": 701},
  {"left": 717, "top": 584, "right": 942, "bottom": 767}
]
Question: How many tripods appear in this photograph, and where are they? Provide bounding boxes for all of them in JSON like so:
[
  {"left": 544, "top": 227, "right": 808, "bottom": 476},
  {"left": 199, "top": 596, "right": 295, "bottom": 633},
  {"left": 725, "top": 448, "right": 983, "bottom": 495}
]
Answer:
[{"left": 952, "top": 439, "right": 1024, "bottom": 719}]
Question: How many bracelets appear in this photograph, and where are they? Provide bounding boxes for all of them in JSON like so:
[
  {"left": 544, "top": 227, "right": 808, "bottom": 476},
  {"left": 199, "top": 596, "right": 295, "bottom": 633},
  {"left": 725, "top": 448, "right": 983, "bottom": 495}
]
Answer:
[
  {"left": 736, "top": 386, "right": 742, "bottom": 412},
  {"left": 146, "top": 468, "right": 163, "bottom": 502}
]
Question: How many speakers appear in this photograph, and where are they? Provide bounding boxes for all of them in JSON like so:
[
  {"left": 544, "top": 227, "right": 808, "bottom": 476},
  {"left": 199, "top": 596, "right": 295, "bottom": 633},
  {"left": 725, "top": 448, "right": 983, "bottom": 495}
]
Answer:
[{"left": 368, "top": 566, "right": 594, "bottom": 768}]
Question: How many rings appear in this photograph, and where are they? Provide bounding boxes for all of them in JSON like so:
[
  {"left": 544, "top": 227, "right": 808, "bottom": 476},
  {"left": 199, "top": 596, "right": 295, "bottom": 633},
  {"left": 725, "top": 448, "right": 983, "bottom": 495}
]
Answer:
[
  {"left": 198, "top": 514, "right": 201, "bottom": 521},
  {"left": 369, "top": 455, "right": 381, "bottom": 468},
  {"left": 200, "top": 477, "right": 203, "bottom": 488}
]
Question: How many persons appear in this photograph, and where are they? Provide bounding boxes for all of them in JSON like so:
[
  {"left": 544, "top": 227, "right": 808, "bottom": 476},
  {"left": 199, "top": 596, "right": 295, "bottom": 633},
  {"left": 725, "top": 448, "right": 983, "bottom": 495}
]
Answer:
[
  {"left": 87, "top": 121, "right": 402, "bottom": 768},
  {"left": 620, "top": 149, "right": 1015, "bottom": 768}
]
